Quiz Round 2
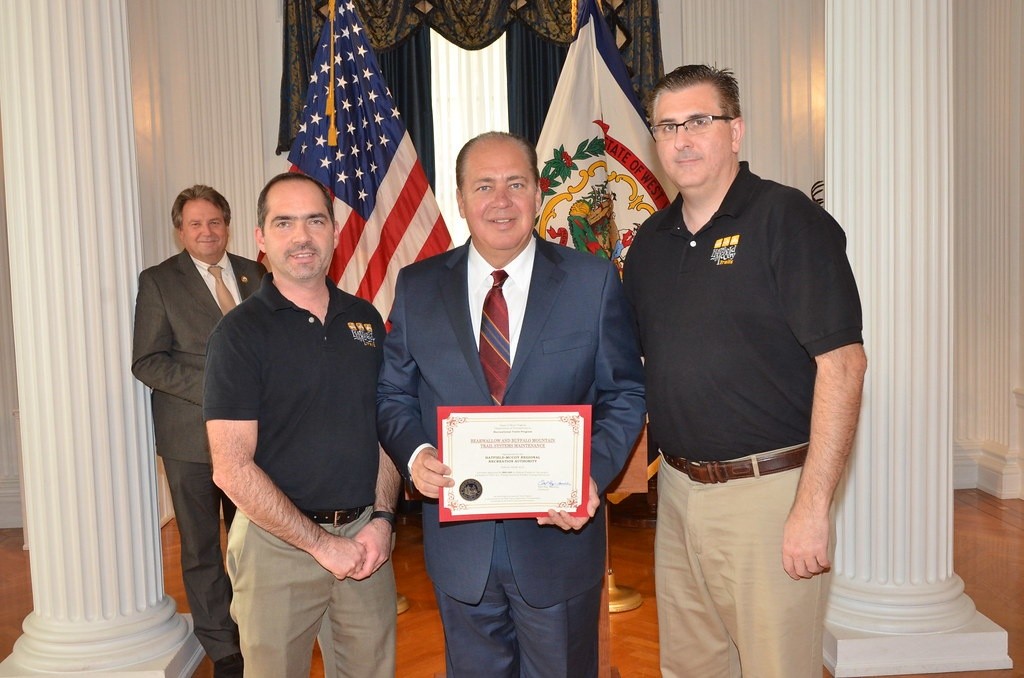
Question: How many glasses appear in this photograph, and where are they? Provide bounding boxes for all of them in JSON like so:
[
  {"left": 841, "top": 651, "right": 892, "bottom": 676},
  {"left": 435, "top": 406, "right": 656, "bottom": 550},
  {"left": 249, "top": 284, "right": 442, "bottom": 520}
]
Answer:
[{"left": 650, "top": 114, "right": 735, "bottom": 140}]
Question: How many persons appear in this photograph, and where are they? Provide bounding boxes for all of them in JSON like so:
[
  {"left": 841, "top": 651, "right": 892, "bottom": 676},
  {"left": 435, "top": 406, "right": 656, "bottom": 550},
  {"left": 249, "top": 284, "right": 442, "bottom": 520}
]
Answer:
[
  {"left": 202, "top": 173, "right": 401, "bottom": 678},
  {"left": 131, "top": 186, "right": 268, "bottom": 678},
  {"left": 376, "top": 131, "right": 616, "bottom": 678},
  {"left": 623, "top": 65, "right": 867, "bottom": 678}
]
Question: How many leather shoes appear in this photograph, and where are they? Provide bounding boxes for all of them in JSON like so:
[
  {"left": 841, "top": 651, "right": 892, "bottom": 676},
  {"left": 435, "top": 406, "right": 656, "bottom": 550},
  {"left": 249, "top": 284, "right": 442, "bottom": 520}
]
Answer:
[{"left": 213, "top": 653, "right": 244, "bottom": 678}]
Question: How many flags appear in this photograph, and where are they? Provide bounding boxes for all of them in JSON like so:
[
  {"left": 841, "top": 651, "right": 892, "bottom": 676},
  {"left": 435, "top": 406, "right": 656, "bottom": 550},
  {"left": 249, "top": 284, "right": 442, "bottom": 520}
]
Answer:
[
  {"left": 256, "top": 0, "right": 454, "bottom": 335},
  {"left": 534, "top": 0, "right": 678, "bottom": 282}
]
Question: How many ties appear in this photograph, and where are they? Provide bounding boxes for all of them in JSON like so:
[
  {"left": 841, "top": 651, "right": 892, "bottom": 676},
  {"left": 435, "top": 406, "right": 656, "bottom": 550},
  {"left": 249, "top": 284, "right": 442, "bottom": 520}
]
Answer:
[
  {"left": 207, "top": 265, "right": 236, "bottom": 317},
  {"left": 478, "top": 270, "right": 511, "bottom": 407}
]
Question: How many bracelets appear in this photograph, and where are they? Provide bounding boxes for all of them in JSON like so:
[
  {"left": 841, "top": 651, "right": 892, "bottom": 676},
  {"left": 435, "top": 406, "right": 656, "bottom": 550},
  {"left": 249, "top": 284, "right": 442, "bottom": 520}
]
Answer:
[{"left": 370, "top": 511, "right": 397, "bottom": 526}]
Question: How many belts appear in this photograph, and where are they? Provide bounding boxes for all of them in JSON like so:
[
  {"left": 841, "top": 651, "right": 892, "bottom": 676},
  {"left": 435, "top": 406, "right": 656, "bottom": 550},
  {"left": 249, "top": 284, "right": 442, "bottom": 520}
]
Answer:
[
  {"left": 297, "top": 509, "right": 366, "bottom": 527},
  {"left": 662, "top": 443, "right": 812, "bottom": 484}
]
